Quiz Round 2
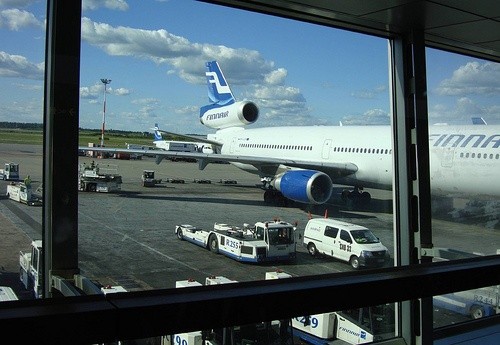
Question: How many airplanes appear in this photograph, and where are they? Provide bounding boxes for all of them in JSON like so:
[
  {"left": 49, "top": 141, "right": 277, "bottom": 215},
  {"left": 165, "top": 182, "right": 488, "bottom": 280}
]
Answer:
[{"left": 79, "top": 60, "right": 500, "bottom": 207}]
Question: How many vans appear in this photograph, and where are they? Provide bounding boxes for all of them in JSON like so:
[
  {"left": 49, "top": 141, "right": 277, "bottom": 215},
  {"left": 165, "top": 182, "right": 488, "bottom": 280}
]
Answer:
[{"left": 303, "top": 217, "right": 391, "bottom": 270}]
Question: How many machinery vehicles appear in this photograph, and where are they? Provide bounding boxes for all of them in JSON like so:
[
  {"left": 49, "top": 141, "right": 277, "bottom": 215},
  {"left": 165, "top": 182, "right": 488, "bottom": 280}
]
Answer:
[{"left": 175, "top": 218, "right": 299, "bottom": 265}]
